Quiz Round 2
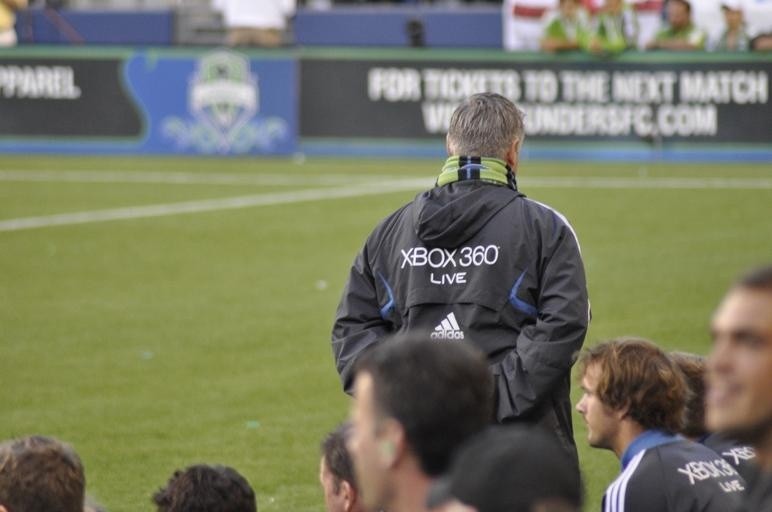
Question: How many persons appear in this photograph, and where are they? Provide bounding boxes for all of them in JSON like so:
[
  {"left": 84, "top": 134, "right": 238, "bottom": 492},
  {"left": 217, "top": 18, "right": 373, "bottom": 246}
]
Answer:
[
  {"left": 349, "top": 333, "right": 493, "bottom": 511},
  {"left": 332, "top": 93, "right": 590, "bottom": 468},
  {"left": 703, "top": 267, "right": 772, "bottom": 511},
  {"left": 570, "top": 332, "right": 749, "bottom": 511},
  {"left": 0, "top": 432, "right": 101, "bottom": 511},
  {"left": 540, "top": 0, "right": 768, "bottom": 54},
  {"left": 425, "top": 423, "right": 582, "bottom": 511},
  {"left": 150, "top": 462, "right": 258, "bottom": 511},
  {"left": 319, "top": 426, "right": 365, "bottom": 511},
  {"left": 1, "top": 0, "right": 29, "bottom": 48},
  {"left": 669, "top": 348, "right": 756, "bottom": 482},
  {"left": 210, "top": 1, "right": 297, "bottom": 49}
]
